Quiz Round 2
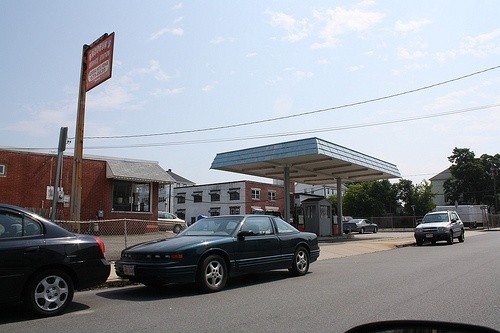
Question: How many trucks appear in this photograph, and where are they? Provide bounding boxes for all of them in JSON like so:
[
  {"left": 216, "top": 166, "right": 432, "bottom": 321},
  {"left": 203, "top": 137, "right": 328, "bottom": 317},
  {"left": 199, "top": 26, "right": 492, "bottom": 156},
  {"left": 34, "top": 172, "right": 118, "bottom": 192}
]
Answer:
[{"left": 434, "top": 204, "right": 490, "bottom": 231}]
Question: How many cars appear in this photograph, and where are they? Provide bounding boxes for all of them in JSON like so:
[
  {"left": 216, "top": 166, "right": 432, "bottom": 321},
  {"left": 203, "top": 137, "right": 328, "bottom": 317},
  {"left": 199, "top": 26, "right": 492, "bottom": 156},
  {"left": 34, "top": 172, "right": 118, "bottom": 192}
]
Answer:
[
  {"left": 0, "top": 202, "right": 112, "bottom": 318},
  {"left": 114, "top": 214, "right": 321, "bottom": 294},
  {"left": 157, "top": 211, "right": 188, "bottom": 234},
  {"left": 342, "top": 218, "right": 379, "bottom": 233}
]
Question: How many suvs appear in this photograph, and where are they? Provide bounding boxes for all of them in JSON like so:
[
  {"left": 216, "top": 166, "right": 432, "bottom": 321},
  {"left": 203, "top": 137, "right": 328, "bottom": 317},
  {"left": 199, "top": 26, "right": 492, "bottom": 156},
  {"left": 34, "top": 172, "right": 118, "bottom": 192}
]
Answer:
[{"left": 413, "top": 210, "right": 466, "bottom": 245}]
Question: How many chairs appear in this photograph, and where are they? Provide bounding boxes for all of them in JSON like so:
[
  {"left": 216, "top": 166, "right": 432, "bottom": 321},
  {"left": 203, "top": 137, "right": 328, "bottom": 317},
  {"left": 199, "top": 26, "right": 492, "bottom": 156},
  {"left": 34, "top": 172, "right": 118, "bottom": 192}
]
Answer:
[
  {"left": 5, "top": 224, "right": 26, "bottom": 237},
  {"left": 246, "top": 225, "right": 259, "bottom": 234},
  {"left": 26, "top": 223, "right": 38, "bottom": 235}
]
